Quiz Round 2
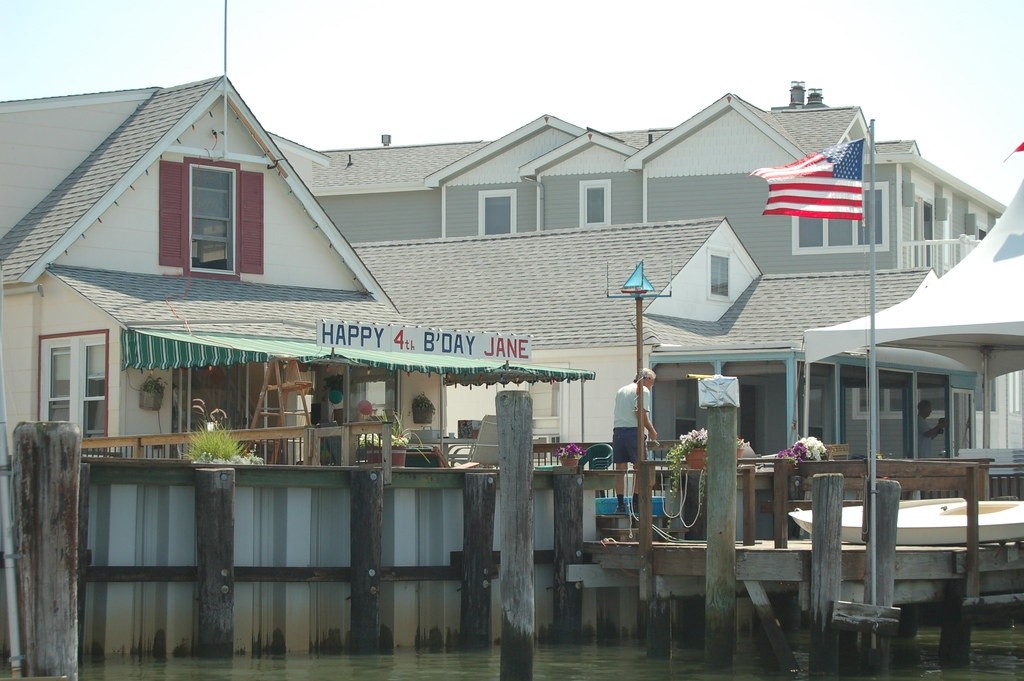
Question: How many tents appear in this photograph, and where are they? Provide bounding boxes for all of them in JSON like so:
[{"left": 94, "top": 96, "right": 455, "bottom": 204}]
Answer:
[{"left": 804, "top": 180, "right": 1024, "bottom": 441}]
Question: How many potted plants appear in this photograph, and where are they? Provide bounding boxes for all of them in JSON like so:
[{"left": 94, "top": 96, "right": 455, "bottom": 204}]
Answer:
[
  {"left": 321, "top": 375, "right": 344, "bottom": 404},
  {"left": 139, "top": 376, "right": 168, "bottom": 411},
  {"left": 407, "top": 392, "right": 436, "bottom": 424}
]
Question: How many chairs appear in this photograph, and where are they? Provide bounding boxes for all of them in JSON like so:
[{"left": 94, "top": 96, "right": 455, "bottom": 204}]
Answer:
[
  {"left": 447, "top": 415, "right": 500, "bottom": 469},
  {"left": 578, "top": 444, "right": 613, "bottom": 498}
]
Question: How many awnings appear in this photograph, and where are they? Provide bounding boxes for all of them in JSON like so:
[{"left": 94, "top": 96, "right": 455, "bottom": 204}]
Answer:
[{"left": 122, "top": 329, "right": 596, "bottom": 388}]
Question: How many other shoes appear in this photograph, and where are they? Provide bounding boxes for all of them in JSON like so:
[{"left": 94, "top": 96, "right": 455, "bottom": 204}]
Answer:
[
  {"left": 614, "top": 504, "right": 626, "bottom": 513},
  {"left": 629, "top": 505, "right": 639, "bottom": 512}
]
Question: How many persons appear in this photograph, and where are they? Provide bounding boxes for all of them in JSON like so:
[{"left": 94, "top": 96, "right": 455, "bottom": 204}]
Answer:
[
  {"left": 917, "top": 400, "right": 946, "bottom": 459},
  {"left": 613, "top": 368, "right": 658, "bottom": 516}
]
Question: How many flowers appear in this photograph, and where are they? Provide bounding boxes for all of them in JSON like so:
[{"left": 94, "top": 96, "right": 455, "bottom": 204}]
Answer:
[
  {"left": 356, "top": 409, "right": 431, "bottom": 464},
  {"left": 668, "top": 428, "right": 708, "bottom": 534},
  {"left": 774, "top": 436, "right": 829, "bottom": 468},
  {"left": 736, "top": 437, "right": 750, "bottom": 448},
  {"left": 554, "top": 444, "right": 587, "bottom": 459}
]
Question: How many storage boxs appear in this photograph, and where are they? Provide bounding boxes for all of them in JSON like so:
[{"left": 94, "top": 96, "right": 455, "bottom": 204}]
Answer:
[
  {"left": 651, "top": 495, "right": 666, "bottom": 515},
  {"left": 596, "top": 497, "right": 631, "bottom": 514},
  {"left": 406, "top": 448, "right": 439, "bottom": 467}
]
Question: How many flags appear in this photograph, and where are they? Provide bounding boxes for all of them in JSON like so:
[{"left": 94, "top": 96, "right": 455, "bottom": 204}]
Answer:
[{"left": 747, "top": 137, "right": 867, "bottom": 227}]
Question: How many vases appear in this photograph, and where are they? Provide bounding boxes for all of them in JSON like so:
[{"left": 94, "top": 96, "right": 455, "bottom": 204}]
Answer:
[
  {"left": 366, "top": 446, "right": 406, "bottom": 467},
  {"left": 561, "top": 459, "right": 579, "bottom": 467},
  {"left": 737, "top": 448, "right": 745, "bottom": 458},
  {"left": 685, "top": 448, "right": 704, "bottom": 469}
]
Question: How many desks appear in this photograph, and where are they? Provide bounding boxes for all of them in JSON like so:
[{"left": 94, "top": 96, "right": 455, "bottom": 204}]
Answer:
[{"left": 436, "top": 438, "right": 477, "bottom": 446}]
